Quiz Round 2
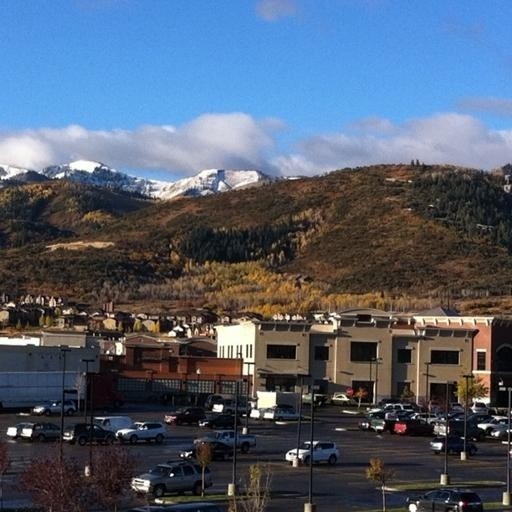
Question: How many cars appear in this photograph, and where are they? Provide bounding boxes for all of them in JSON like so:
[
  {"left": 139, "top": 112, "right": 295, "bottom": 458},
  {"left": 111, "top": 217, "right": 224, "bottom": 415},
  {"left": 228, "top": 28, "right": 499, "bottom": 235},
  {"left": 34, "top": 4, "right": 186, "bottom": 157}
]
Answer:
[
  {"left": 364, "top": 398, "right": 512, "bottom": 456},
  {"left": 129, "top": 459, "right": 215, "bottom": 499},
  {"left": 19, "top": 421, "right": 66, "bottom": 442},
  {"left": 61, "top": 422, "right": 117, "bottom": 446},
  {"left": 115, "top": 421, "right": 167, "bottom": 445},
  {"left": 177, "top": 441, "right": 236, "bottom": 462},
  {"left": 33, "top": 398, "right": 78, "bottom": 418},
  {"left": 403, "top": 486, "right": 484, "bottom": 512},
  {"left": 284, "top": 439, "right": 341, "bottom": 467},
  {"left": 164, "top": 406, "right": 207, "bottom": 426},
  {"left": 211, "top": 397, "right": 254, "bottom": 418},
  {"left": 6, "top": 421, "right": 38, "bottom": 438},
  {"left": 198, "top": 413, "right": 241, "bottom": 429}
]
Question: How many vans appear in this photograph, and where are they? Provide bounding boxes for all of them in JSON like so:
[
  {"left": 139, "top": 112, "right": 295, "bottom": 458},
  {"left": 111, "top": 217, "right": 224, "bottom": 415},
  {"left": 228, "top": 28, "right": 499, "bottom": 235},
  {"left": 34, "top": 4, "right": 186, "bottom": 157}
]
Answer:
[{"left": 88, "top": 415, "right": 135, "bottom": 434}]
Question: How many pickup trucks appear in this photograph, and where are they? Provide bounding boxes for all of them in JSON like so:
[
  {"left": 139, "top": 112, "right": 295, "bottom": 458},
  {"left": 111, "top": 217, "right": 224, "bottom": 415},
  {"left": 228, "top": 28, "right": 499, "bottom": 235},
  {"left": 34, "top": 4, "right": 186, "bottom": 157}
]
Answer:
[{"left": 193, "top": 428, "right": 257, "bottom": 455}]
